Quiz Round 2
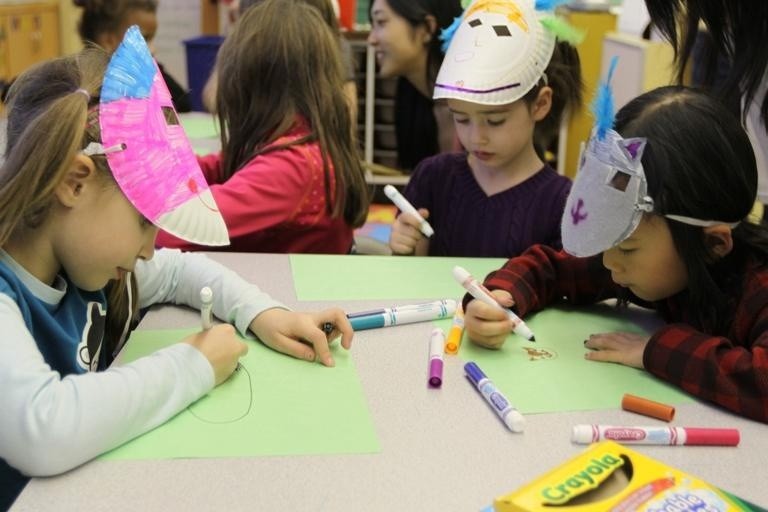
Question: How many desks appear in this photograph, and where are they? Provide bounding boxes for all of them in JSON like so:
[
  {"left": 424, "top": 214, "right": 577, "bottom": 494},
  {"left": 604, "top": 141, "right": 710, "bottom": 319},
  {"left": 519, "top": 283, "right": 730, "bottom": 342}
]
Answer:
[{"left": 8, "top": 251, "right": 768, "bottom": 512}]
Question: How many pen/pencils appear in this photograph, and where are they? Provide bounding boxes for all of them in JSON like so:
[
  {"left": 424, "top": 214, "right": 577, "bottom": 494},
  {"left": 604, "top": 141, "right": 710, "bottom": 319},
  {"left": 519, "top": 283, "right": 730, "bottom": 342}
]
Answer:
[
  {"left": 345, "top": 299, "right": 457, "bottom": 318},
  {"left": 428, "top": 326, "right": 447, "bottom": 387},
  {"left": 200, "top": 287, "right": 214, "bottom": 331},
  {"left": 464, "top": 361, "right": 527, "bottom": 433},
  {"left": 444, "top": 302, "right": 465, "bottom": 354},
  {"left": 383, "top": 184, "right": 436, "bottom": 239},
  {"left": 570, "top": 422, "right": 740, "bottom": 446},
  {"left": 348, "top": 302, "right": 458, "bottom": 331},
  {"left": 451, "top": 264, "right": 536, "bottom": 341}
]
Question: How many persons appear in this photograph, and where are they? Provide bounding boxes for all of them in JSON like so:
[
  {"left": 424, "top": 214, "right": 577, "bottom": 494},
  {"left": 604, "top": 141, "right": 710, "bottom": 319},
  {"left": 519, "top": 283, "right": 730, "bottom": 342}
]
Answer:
[
  {"left": 202, "top": 0, "right": 357, "bottom": 135},
  {"left": 388, "top": 0, "right": 574, "bottom": 256},
  {"left": 0, "top": 48, "right": 354, "bottom": 512},
  {"left": 462, "top": 85, "right": 768, "bottom": 424},
  {"left": 644, "top": 0, "right": 768, "bottom": 207},
  {"left": 72, "top": 1, "right": 191, "bottom": 113},
  {"left": 154, "top": 0, "right": 368, "bottom": 257},
  {"left": 367, "top": 1, "right": 465, "bottom": 257}
]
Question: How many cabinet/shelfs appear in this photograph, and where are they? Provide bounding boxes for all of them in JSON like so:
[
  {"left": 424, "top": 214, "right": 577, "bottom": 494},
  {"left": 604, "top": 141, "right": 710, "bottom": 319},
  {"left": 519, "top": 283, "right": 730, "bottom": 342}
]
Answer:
[{"left": 0, "top": 1, "right": 62, "bottom": 86}]
